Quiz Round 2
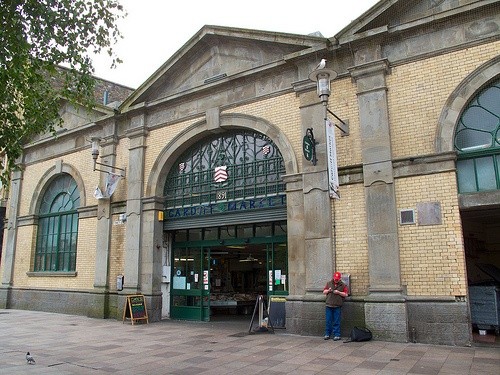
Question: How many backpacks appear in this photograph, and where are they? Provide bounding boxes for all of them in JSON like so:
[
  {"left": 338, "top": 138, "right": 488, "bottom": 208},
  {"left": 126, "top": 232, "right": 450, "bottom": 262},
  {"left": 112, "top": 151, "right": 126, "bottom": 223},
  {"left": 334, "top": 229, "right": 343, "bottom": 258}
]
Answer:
[{"left": 351, "top": 326, "right": 372, "bottom": 342}]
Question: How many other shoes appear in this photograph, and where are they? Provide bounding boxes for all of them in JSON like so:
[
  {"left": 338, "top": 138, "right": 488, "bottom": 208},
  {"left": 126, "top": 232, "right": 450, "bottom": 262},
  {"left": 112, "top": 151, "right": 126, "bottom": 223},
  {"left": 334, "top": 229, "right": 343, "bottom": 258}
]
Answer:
[
  {"left": 324, "top": 335, "right": 330, "bottom": 340},
  {"left": 333, "top": 336, "right": 341, "bottom": 340}
]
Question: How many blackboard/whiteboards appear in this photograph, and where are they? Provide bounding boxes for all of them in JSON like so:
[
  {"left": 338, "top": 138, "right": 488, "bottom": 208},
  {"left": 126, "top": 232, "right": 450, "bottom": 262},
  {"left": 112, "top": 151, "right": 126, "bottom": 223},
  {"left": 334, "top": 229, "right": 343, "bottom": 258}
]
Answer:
[{"left": 123, "top": 295, "right": 147, "bottom": 320}]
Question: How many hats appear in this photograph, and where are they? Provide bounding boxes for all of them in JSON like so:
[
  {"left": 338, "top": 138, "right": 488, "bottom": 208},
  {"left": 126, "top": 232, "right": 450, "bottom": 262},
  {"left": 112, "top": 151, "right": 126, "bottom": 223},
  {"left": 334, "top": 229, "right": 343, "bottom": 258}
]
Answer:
[{"left": 334, "top": 272, "right": 341, "bottom": 280}]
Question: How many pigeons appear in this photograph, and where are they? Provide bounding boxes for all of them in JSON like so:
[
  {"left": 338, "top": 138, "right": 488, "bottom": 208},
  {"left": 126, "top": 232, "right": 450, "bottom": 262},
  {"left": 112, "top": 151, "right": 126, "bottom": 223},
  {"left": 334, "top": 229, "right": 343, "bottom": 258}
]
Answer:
[{"left": 25, "top": 351, "right": 36, "bottom": 364}]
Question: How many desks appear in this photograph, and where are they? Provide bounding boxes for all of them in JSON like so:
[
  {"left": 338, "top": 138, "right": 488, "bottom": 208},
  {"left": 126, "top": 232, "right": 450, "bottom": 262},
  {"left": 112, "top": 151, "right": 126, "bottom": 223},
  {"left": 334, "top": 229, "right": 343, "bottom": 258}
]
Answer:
[{"left": 199, "top": 299, "right": 255, "bottom": 314}]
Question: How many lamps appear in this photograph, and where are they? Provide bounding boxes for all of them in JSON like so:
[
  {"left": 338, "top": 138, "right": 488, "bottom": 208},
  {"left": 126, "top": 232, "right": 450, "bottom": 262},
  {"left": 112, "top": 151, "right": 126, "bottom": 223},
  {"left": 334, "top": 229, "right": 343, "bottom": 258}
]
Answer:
[
  {"left": 88, "top": 136, "right": 125, "bottom": 179},
  {"left": 308, "top": 69, "right": 349, "bottom": 132}
]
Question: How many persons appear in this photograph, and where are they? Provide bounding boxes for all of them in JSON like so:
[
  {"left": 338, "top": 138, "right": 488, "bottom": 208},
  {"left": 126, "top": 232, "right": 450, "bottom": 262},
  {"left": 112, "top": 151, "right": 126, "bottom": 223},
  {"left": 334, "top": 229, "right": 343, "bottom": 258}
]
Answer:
[{"left": 323, "top": 272, "right": 348, "bottom": 341}]
